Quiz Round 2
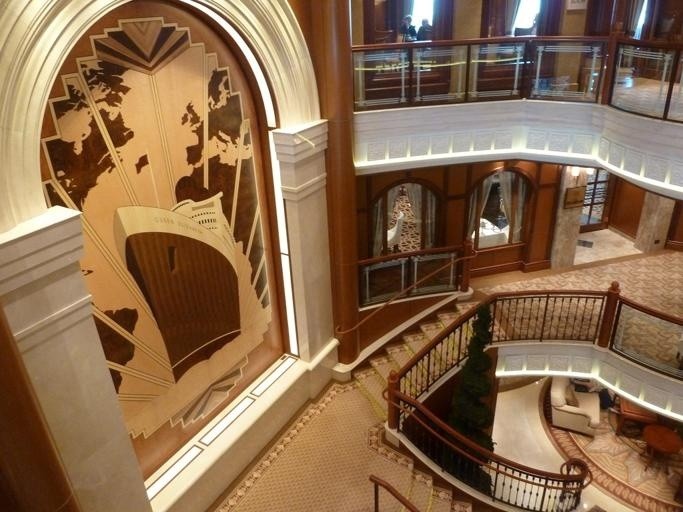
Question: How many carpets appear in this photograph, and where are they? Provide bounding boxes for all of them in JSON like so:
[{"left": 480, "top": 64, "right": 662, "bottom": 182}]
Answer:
[{"left": 537, "top": 376, "right": 683, "bottom": 512}]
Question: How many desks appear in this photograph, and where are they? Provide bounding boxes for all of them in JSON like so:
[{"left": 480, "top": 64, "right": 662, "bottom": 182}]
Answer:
[
  {"left": 611, "top": 392, "right": 659, "bottom": 438},
  {"left": 638, "top": 424, "right": 683, "bottom": 476},
  {"left": 471, "top": 215, "right": 508, "bottom": 250}
]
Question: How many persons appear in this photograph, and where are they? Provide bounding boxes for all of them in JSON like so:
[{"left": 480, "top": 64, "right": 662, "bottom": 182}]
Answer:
[
  {"left": 398, "top": 15, "right": 416, "bottom": 38},
  {"left": 416, "top": 19, "right": 432, "bottom": 40}
]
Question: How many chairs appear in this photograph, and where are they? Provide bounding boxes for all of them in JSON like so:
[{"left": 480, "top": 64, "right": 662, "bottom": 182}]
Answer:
[
  {"left": 375, "top": 26, "right": 432, "bottom": 75},
  {"left": 550, "top": 376, "right": 602, "bottom": 438},
  {"left": 387, "top": 210, "right": 404, "bottom": 253}
]
